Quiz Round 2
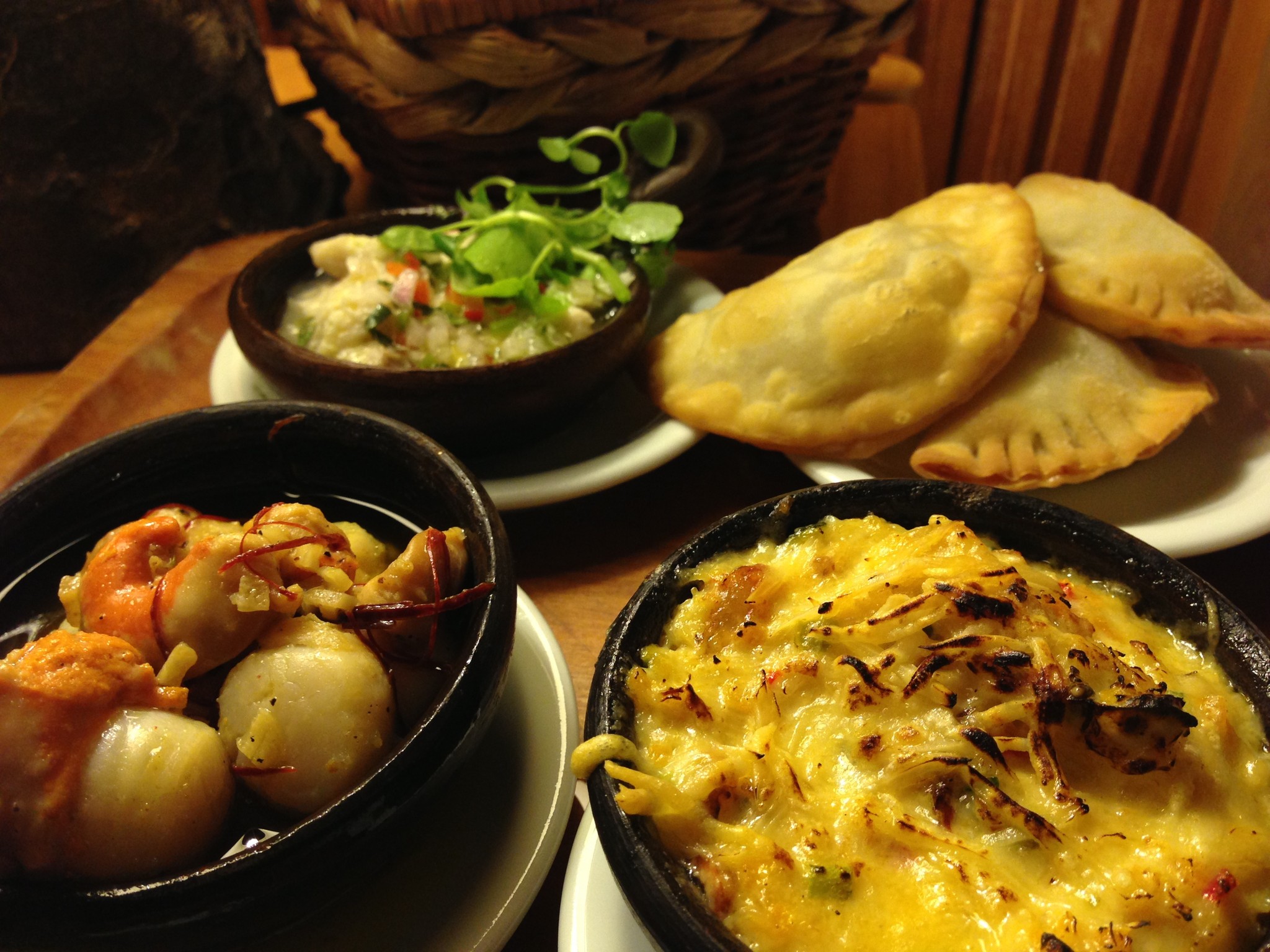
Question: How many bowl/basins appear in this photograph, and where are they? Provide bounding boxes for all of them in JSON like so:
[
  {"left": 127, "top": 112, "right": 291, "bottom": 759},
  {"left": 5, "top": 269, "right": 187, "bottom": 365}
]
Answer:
[
  {"left": 0, "top": 398, "right": 516, "bottom": 939},
  {"left": 583, "top": 475, "right": 1270, "bottom": 952},
  {"left": 225, "top": 204, "right": 652, "bottom": 435}
]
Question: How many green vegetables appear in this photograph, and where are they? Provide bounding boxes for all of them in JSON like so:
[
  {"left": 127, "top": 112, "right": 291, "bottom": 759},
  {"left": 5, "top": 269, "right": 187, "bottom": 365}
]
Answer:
[{"left": 367, "top": 113, "right": 683, "bottom": 333}]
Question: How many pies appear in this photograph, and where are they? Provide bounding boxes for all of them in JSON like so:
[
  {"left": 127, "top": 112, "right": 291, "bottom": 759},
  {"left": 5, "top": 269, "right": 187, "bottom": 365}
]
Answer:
[
  {"left": 635, "top": 180, "right": 1044, "bottom": 461},
  {"left": 911, "top": 312, "right": 1220, "bottom": 490},
  {"left": 1013, "top": 169, "right": 1270, "bottom": 356}
]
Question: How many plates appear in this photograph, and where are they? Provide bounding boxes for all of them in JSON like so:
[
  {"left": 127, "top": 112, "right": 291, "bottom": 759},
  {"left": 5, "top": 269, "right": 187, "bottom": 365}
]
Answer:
[
  {"left": 205, "top": 260, "right": 727, "bottom": 508},
  {"left": 556, "top": 808, "right": 655, "bottom": 950},
  {"left": 0, "top": 569, "right": 582, "bottom": 951},
  {"left": 775, "top": 346, "right": 1269, "bottom": 561}
]
